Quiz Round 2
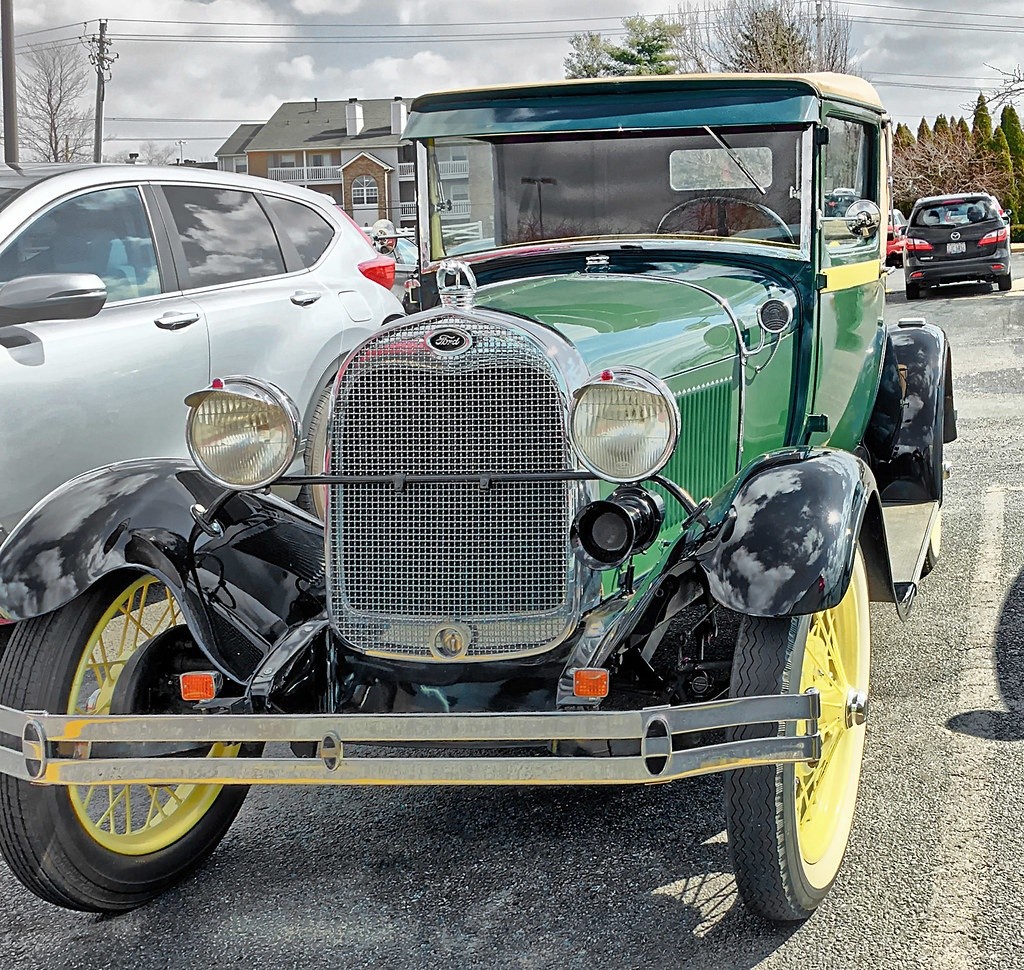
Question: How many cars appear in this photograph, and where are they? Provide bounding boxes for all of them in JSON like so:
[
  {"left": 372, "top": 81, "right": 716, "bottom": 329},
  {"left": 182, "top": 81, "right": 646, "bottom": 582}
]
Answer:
[
  {"left": 0, "top": 163, "right": 408, "bottom": 543},
  {"left": 888, "top": 209, "right": 907, "bottom": 225},
  {"left": 887, "top": 225, "right": 908, "bottom": 255},
  {"left": 0, "top": 74, "right": 959, "bottom": 920},
  {"left": 401, "top": 237, "right": 499, "bottom": 314},
  {"left": 833, "top": 188, "right": 855, "bottom": 195},
  {"left": 897, "top": 192, "right": 1012, "bottom": 300},
  {"left": 824, "top": 194, "right": 860, "bottom": 217},
  {"left": 945, "top": 196, "right": 1012, "bottom": 243},
  {"left": 360, "top": 225, "right": 419, "bottom": 302}
]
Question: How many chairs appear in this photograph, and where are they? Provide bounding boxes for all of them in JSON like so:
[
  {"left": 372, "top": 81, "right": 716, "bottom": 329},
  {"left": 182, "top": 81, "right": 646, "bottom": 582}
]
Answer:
[
  {"left": 23, "top": 221, "right": 132, "bottom": 297},
  {"left": 967, "top": 206, "right": 983, "bottom": 222},
  {"left": 929, "top": 211, "right": 940, "bottom": 224}
]
